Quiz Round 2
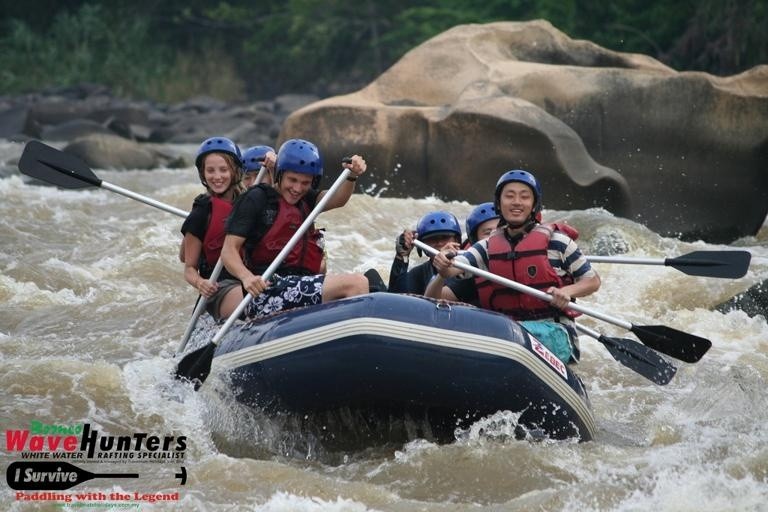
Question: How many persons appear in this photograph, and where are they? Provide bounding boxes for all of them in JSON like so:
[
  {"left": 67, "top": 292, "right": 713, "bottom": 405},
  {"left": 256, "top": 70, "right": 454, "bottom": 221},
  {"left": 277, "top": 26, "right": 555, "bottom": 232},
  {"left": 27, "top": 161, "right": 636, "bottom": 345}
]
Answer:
[
  {"left": 241, "top": 144, "right": 275, "bottom": 190},
  {"left": 387, "top": 212, "right": 463, "bottom": 298},
  {"left": 424, "top": 169, "right": 600, "bottom": 367},
  {"left": 180, "top": 136, "right": 278, "bottom": 324},
  {"left": 217, "top": 139, "right": 372, "bottom": 323},
  {"left": 439, "top": 202, "right": 504, "bottom": 309}
]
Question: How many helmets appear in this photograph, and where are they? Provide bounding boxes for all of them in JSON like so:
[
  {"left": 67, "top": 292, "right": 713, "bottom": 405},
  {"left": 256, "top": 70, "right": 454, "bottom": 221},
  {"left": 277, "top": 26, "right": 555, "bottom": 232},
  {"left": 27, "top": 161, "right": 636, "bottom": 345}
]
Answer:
[
  {"left": 273, "top": 139, "right": 323, "bottom": 189},
  {"left": 415, "top": 212, "right": 462, "bottom": 256},
  {"left": 493, "top": 170, "right": 540, "bottom": 214},
  {"left": 242, "top": 145, "right": 275, "bottom": 171},
  {"left": 196, "top": 137, "right": 243, "bottom": 169},
  {"left": 466, "top": 203, "right": 502, "bottom": 245}
]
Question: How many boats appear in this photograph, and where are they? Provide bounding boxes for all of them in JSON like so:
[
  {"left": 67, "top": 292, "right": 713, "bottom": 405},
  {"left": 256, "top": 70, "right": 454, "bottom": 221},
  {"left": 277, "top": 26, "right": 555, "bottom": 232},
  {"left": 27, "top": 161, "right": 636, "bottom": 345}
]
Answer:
[{"left": 185, "top": 290, "right": 600, "bottom": 459}]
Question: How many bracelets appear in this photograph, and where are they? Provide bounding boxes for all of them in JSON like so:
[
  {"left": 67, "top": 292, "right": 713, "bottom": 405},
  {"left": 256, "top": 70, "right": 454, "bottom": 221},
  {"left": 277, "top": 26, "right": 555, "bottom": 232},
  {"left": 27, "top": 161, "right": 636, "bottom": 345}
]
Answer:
[{"left": 346, "top": 175, "right": 358, "bottom": 181}]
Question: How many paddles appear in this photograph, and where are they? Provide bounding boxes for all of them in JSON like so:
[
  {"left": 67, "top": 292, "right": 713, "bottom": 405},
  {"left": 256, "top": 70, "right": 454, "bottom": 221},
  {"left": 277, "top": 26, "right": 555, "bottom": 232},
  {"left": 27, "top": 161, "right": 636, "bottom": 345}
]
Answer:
[
  {"left": 574, "top": 322, "right": 678, "bottom": 388},
  {"left": 411, "top": 238, "right": 714, "bottom": 365},
  {"left": 586, "top": 250, "right": 752, "bottom": 280},
  {"left": 174, "top": 155, "right": 357, "bottom": 393},
  {"left": 16, "top": 138, "right": 192, "bottom": 220}
]
